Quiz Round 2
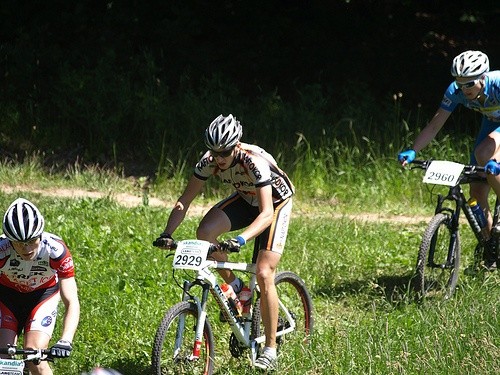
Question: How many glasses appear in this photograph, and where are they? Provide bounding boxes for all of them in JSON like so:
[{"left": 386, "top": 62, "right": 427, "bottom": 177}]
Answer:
[
  {"left": 210, "top": 147, "right": 235, "bottom": 158},
  {"left": 11, "top": 237, "right": 39, "bottom": 247},
  {"left": 456, "top": 77, "right": 481, "bottom": 89}
]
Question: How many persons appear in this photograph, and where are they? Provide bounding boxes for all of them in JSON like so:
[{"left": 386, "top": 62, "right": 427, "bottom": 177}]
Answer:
[
  {"left": 0, "top": 198, "right": 80, "bottom": 375},
  {"left": 157, "top": 113, "right": 295, "bottom": 373},
  {"left": 399, "top": 51, "right": 500, "bottom": 261}
]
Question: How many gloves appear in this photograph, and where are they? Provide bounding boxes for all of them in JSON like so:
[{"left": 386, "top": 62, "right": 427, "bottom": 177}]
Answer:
[{"left": 50, "top": 340, "right": 72, "bottom": 358}]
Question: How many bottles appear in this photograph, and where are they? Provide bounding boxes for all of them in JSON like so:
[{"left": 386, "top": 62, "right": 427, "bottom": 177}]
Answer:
[
  {"left": 468, "top": 197, "right": 488, "bottom": 228},
  {"left": 239, "top": 286, "right": 251, "bottom": 316},
  {"left": 220, "top": 283, "right": 243, "bottom": 314}
]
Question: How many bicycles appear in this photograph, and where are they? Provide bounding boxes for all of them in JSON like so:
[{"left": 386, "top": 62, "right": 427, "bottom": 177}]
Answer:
[
  {"left": 402, "top": 156, "right": 500, "bottom": 310},
  {"left": 0, "top": 344, "right": 54, "bottom": 375},
  {"left": 152, "top": 239, "right": 316, "bottom": 375}
]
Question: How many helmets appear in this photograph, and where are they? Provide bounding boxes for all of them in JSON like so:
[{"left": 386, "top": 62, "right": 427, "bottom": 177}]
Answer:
[
  {"left": 450, "top": 50, "right": 490, "bottom": 77},
  {"left": 2, "top": 198, "right": 44, "bottom": 242},
  {"left": 204, "top": 113, "right": 243, "bottom": 151}
]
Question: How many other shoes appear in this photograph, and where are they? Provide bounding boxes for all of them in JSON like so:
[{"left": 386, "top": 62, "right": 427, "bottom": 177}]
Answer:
[
  {"left": 492, "top": 205, "right": 500, "bottom": 232},
  {"left": 464, "top": 262, "right": 497, "bottom": 276},
  {"left": 253, "top": 351, "right": 277, "bottom": 371},
  {"left": 219, "top": 278, "right": 247, "bottom": 322}
]
